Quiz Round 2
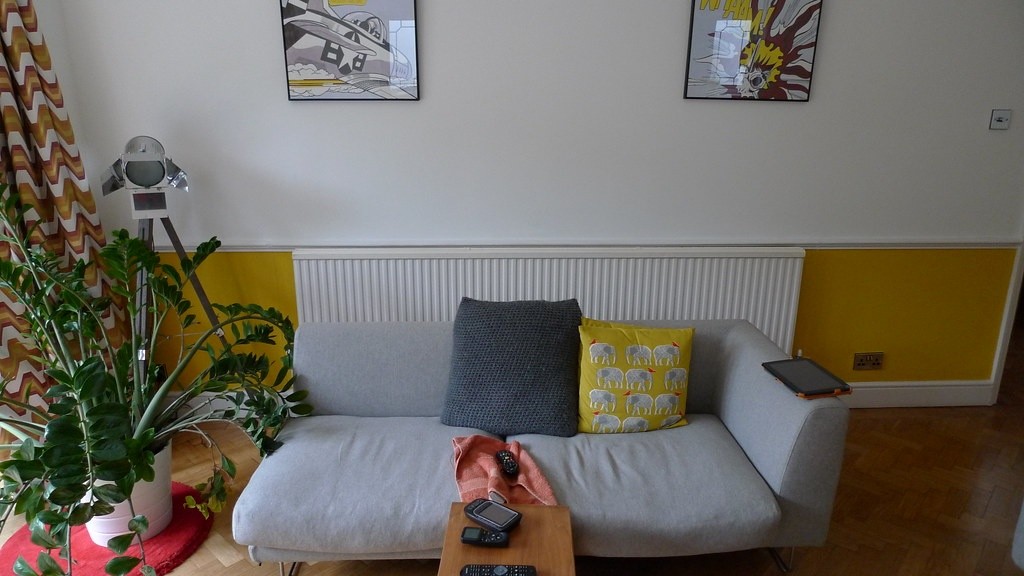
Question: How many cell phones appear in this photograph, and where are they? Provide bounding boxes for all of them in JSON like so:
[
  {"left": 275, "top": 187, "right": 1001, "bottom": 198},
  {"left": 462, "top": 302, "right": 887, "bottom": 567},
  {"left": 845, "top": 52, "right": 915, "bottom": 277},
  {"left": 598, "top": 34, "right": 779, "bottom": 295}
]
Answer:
[{"left": 460, "top": 498, "right": 522, "bottom": 547}]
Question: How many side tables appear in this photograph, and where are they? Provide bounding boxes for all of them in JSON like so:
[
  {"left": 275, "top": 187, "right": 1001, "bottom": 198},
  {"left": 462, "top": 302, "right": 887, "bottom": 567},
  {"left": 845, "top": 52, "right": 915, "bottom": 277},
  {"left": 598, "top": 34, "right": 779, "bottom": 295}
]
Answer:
[{"left": 435, "top": 499, "right": 577, "bottom": 576}]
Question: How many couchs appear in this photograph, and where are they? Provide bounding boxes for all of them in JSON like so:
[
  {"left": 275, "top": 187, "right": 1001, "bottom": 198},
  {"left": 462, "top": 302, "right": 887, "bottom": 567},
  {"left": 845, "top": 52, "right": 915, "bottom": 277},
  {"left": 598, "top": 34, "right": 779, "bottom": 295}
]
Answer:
[{"left": 231, "top": 317, "right": 853, "bottom": 576}]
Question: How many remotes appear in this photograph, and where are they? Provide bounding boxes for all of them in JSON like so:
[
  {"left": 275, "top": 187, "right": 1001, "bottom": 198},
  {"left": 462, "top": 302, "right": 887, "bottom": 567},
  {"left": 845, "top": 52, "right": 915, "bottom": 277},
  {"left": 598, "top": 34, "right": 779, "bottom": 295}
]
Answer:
[
  {"left": 460, "top": 564, "right": 536, "bottom": 576},
  {"left": 497, "top": 449, "right": 519, "bottom": 477}
]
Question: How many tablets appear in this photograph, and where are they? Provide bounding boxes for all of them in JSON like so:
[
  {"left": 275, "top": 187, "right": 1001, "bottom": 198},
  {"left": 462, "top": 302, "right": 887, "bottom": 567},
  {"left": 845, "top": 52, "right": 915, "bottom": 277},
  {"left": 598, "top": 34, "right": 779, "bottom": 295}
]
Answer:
[{"left": 761, "top": 358, "right": 852, "bottom": 399}]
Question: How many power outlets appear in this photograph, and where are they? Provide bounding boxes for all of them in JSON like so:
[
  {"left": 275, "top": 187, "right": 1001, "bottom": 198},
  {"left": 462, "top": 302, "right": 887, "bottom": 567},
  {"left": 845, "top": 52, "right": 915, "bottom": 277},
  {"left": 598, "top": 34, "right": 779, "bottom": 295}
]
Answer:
[{"left": 853, "top": 352, "right": 884, "bottom": 371}]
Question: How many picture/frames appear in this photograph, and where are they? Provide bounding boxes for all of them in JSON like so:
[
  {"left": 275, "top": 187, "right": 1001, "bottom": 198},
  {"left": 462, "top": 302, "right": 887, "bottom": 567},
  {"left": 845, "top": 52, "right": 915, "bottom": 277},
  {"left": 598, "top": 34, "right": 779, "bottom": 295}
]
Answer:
[
  {"left": 280, "top": 0, "right": 422, "bottom": 101},
  {"left": 680, "top": 0, "right": 823, "bottom": 104}
]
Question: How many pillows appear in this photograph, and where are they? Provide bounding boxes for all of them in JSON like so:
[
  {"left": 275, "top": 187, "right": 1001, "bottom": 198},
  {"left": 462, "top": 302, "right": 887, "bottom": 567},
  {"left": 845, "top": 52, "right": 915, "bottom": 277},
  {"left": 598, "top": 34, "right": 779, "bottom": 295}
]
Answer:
[
  {"left": 440, "top": 295, "right": 584, "bottom": 441},
  {"left": 571, "top": 323, "right": 696, "bottom": 435}
]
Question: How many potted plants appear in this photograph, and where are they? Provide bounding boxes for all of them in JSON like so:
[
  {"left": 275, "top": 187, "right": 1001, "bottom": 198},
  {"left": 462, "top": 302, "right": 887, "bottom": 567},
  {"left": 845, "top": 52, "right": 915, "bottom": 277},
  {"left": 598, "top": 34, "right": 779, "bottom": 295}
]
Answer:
[{"left": 0, "top": 186, "right": 313, "bottom": 575}]
